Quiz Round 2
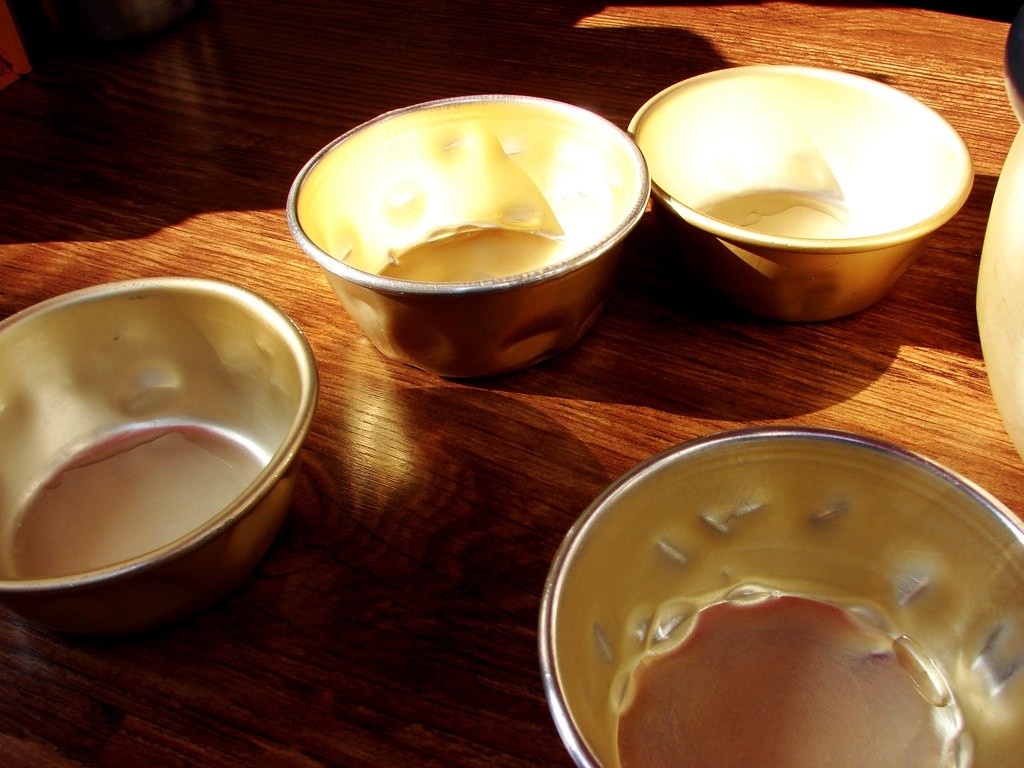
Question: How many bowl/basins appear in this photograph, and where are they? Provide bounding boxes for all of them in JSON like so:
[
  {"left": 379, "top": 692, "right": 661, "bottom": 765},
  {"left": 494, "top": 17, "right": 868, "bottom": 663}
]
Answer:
[
  {"left": 629, "top": 62, "right": 975, "bottom": 325},
  {"left": 0, "top": 275, "right": 318, "bottom": 651},
  {"left": 285, "top": 92, "right": 647, "bottom": 382},
  {"left": 536, "top": 424, "right": 1024, "bottom": 768}
]
